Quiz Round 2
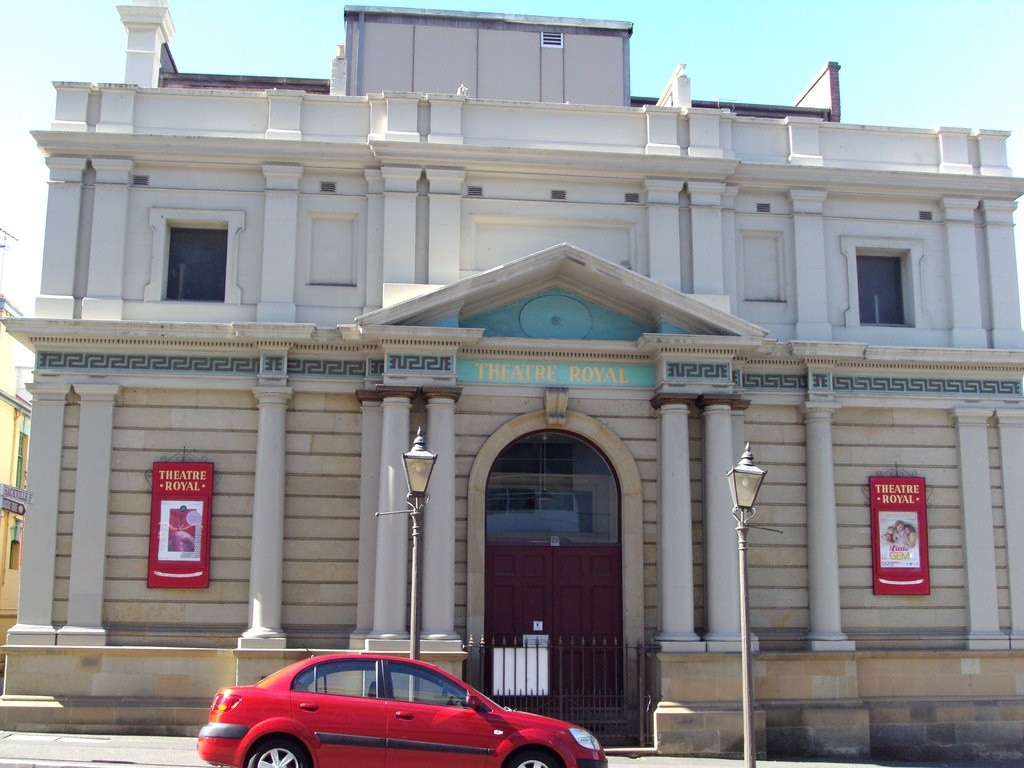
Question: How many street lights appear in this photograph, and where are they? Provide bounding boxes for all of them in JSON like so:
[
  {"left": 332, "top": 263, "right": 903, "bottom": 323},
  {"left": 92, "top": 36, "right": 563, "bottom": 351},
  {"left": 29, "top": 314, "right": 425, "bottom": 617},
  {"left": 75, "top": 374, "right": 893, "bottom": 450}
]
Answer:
[
  {"left": 401, "top": 425, "right": 439, "bottom": 704},
  {"left": 727, "top": 441, "right": 767, "bottom": 768}
]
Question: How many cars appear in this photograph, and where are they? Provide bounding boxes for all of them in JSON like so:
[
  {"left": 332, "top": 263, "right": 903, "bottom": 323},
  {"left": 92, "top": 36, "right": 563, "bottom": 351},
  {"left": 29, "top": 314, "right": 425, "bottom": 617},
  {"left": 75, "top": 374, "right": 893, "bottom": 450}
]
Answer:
[{"left": 194, "top": 655, "right": 609, "bottom": 768}]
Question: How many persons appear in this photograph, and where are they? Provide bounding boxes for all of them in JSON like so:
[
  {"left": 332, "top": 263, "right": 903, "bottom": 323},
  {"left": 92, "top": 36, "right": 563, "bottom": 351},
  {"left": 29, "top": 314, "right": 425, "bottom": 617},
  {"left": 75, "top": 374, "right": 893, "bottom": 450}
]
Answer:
[{"left": 883, "top": 520, "right": 916, "bottom": 547}]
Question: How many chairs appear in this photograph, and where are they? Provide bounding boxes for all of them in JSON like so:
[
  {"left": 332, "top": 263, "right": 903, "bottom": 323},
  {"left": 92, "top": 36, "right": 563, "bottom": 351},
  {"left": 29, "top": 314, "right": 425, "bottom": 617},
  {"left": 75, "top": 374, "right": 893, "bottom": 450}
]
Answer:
[{"left": 368, "top": 681, "right": 376, "bottom": 697}]
